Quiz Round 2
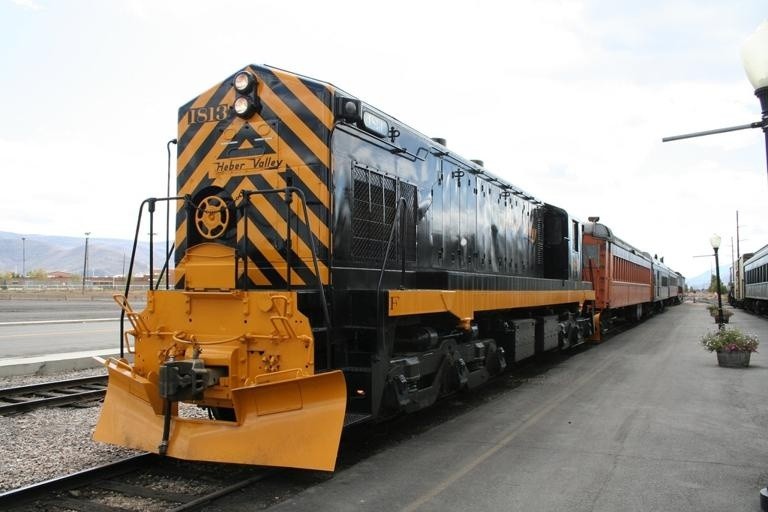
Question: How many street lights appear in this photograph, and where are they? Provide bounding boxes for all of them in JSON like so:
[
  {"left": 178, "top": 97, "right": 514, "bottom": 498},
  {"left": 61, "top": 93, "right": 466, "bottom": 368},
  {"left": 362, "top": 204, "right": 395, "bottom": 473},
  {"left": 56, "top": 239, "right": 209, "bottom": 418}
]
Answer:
[
  {"left": 691, "top": 232, "right": 729, "bottom": 330},
  {"left": 652, "top": 20, "right": 768, "bottom": 284},
  {"left": 79, "top": 231, "right": 92, "bottom": 294},
  {"left": 21, "top": 237, "right": 27, "bottom": 276}
]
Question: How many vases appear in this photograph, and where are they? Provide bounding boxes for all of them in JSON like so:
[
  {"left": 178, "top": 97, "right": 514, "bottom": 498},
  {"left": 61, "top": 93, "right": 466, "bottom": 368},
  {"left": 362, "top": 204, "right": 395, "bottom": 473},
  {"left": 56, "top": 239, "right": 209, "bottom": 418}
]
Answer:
[
  {"left": 715, "top": 316, "right": 729, "bottom": 323},
  {"left": 715, "top": 345, "right": 751, "bottom": 369}
]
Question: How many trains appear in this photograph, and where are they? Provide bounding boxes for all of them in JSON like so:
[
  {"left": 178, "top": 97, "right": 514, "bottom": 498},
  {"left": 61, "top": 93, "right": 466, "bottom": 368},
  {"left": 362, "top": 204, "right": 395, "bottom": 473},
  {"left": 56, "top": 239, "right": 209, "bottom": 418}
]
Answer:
[
  {"left": 88, "top": 54, "right": 685, "bottom": 472},
  {"left": 728, "top": 241, "right": 767, "bottom": 320}
]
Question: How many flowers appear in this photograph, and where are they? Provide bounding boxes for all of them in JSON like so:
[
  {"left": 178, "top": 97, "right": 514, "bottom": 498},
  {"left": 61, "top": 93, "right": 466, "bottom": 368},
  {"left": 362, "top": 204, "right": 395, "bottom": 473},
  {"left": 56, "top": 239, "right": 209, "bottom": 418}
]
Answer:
[
  {"left": 708, "top": 303, "right": 734, "bottom": 317},
  {"left": 695, "top": 323, "right": 760, "bottom": 353}
]
